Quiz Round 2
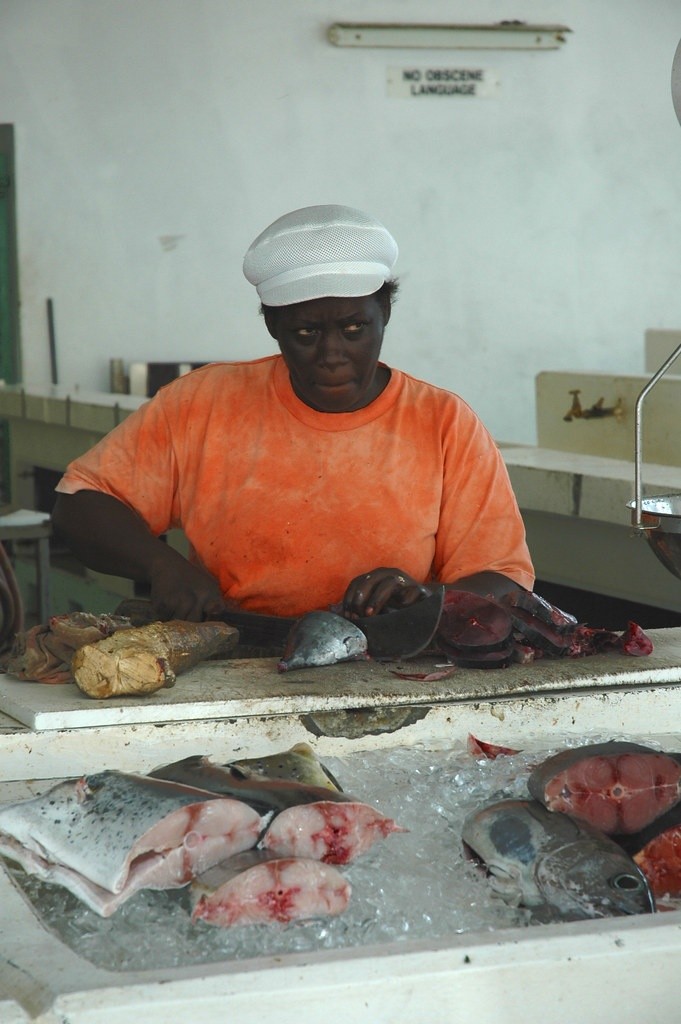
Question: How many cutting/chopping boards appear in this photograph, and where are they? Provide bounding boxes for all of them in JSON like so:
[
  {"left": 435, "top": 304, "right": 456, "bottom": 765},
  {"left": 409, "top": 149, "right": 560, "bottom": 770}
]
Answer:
[{"left": 0, "top": 626, "right": 680, "bottom": 731}]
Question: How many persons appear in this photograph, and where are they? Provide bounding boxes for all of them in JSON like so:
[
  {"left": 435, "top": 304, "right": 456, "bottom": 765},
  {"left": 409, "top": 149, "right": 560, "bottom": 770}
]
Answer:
[{"left": 53, "top": 203, "right": 538, "bottom": 628}]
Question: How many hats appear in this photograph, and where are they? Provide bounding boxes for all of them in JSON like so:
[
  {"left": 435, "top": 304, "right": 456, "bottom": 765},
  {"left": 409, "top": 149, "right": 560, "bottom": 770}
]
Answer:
[{"left": 242, "top": 204, "right": 399, "bottom": 307}]
